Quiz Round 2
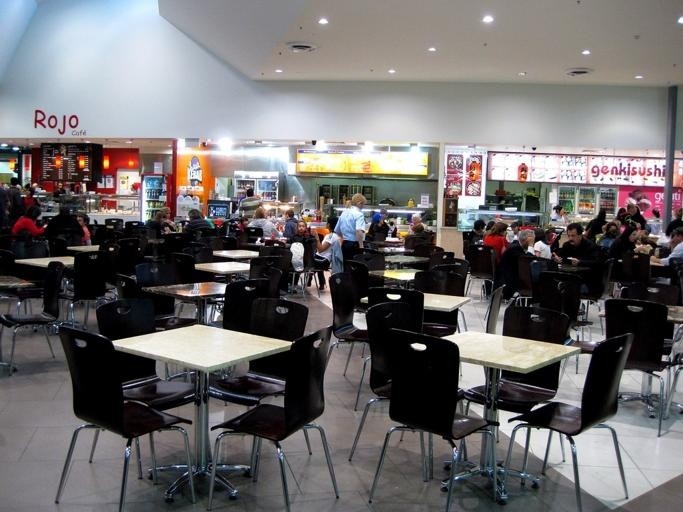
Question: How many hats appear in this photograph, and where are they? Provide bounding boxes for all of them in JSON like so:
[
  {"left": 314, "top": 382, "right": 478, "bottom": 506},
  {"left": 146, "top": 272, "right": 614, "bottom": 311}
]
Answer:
[
  {"left": 327, "top": 216, "right": 338, "bottom": 225},
  {"left": 372, "top": 213, "right": 385, "bottom": 222},
  {"left": 410, "top": 223, "right": 424, "bottom": 232}
]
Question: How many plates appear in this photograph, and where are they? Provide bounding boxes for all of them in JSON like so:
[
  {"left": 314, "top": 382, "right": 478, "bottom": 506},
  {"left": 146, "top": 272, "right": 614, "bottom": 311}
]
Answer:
[{"left": 287, "top": 202, "right": 298, "bottom": 205}]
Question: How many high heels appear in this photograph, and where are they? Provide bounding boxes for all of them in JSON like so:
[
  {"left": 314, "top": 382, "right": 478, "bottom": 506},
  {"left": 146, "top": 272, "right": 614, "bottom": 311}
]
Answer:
[
  {"left": 319, "top": 280, "right": 327, "bottom": 290},
  {"left": 307, "top": 276, "right": 313, "bottom": 286}
]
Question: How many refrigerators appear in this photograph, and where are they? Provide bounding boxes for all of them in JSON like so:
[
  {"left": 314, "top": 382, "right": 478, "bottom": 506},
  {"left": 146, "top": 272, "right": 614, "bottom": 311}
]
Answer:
[
  {"left": 140, "top": 173, "right": 175, "bottom": 224},
  {"left": 538, "top": 182, "right": 617, "bottom": 227}
]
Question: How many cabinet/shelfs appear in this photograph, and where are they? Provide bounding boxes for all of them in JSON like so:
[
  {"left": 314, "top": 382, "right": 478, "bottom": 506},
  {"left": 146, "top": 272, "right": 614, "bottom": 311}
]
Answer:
[{"left": 553, "top": 182, "right": 618, "bottom": 217}]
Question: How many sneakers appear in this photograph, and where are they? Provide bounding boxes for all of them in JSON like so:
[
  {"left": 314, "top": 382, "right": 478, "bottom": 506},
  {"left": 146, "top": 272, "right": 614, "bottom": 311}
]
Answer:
[{"left": 353, "top": 303, "right": 369, "bottom": 313}]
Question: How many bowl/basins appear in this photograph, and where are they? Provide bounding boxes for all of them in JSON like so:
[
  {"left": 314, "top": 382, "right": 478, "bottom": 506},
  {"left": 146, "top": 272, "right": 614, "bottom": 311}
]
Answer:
[{"left": 504, "top": 207, "right": 517, "bottom": 212}]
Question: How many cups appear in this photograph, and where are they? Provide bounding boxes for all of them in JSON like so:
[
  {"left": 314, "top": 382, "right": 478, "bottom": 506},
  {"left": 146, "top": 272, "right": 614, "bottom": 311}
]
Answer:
[{"left": 292, "top": 195, "right": 297, "bottom": 202}]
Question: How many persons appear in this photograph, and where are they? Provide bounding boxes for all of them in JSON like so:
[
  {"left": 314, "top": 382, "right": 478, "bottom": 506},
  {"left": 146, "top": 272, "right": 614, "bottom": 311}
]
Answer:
[
  {"left": 146, "top": 187, "right": 338, "bottom": 296},
  {"left": 466, "top": 200, "right": 683, "bottom": 319},
  {"left": 288, "top": 193, "right": 435, "bottom": 295},
  {"left": 1, "top": 175, "right": 92, "bottom": 257}
]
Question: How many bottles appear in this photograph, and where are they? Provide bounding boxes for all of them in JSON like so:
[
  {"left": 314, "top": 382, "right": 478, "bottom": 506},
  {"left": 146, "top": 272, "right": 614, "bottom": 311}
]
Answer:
[
  {"left": 559, "top": 188, "right": 614, "bottom": 215},
  {"left": 145, "top": 188, "right": 164, "bottom": 219},
  {"left": 236, "top": 180, "right": 276, "bottom": 200}
]
